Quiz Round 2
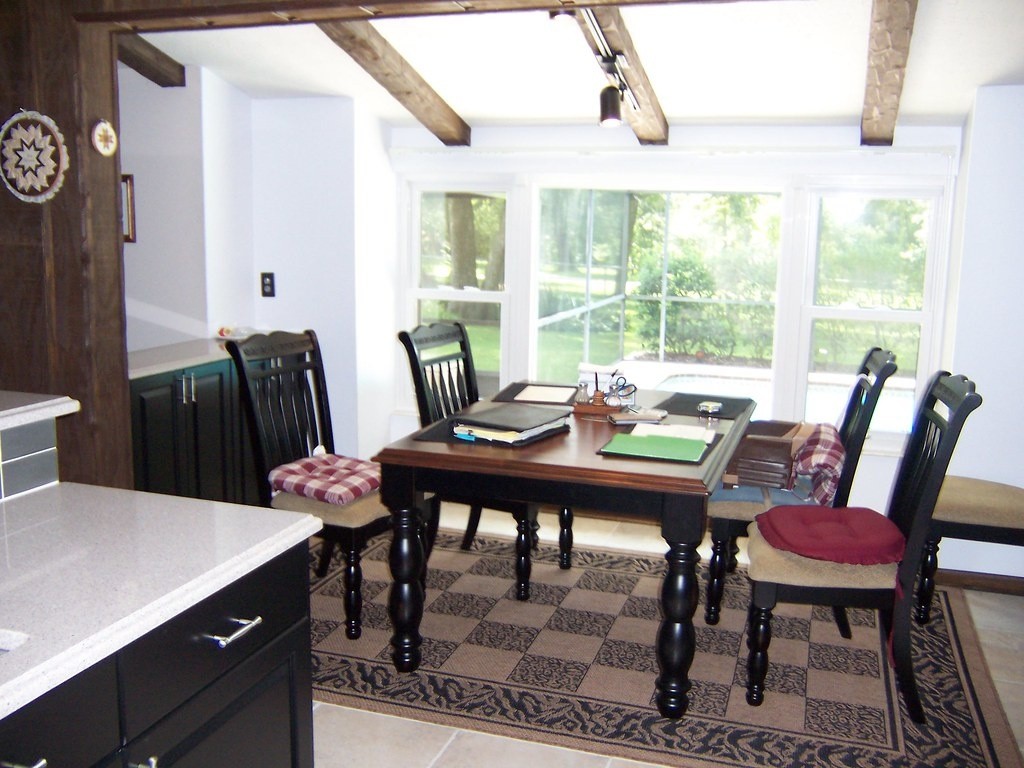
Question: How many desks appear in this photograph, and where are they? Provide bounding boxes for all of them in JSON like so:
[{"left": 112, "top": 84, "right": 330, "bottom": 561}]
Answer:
[{"left": 369, "top": 378, "right": 759, "bottom": 721}]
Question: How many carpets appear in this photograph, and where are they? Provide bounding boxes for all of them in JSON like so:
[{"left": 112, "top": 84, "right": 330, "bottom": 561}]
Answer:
[{"left": 308, "top": 525, "right": 1024, "bottom": 768}]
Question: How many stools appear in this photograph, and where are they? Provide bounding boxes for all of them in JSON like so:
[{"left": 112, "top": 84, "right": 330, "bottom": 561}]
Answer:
[{"left": 913, "top": 474, "right": 1024, "bottom": 626}]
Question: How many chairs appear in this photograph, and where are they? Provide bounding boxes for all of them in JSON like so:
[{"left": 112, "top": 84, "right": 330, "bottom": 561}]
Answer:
[
  {"left": 704, "top": 345, "right": 899, "bottom": 640},
  {"left": 745, "top": 368, "right": 984, "bottom": 725},
  {"left": 397, "top": 320, "right": 542, "bottom": 601},
  {"left": 224, "top": 327, "right": 435, "bottom": 642}
]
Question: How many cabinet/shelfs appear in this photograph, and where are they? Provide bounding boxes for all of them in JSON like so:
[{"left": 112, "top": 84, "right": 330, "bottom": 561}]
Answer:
[
  {"left": 0, "top": 480, "right": 324, "bottom": 768},
  {"left": 126, "top": 338, "right": 261, "bottom": 509}
]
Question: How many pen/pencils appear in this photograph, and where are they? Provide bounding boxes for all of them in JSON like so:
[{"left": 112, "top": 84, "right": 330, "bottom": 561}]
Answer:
[{"left": 594, "top": 371, "right": 599, "bottom": 391}]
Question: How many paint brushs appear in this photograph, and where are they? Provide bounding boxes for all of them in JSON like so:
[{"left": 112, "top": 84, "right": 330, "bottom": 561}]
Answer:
[{"left": 600, "top": 368, "right": 619, "bottom": 393}]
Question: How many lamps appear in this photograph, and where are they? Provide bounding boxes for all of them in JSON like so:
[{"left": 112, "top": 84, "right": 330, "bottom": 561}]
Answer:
[{"left": 597, "top": 82, "right": 627, "bottom": 130}]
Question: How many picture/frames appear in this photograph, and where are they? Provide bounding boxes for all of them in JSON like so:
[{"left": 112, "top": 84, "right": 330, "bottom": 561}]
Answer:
[{"left": 121, "top": 173, "right": 137, "bottom": 244}]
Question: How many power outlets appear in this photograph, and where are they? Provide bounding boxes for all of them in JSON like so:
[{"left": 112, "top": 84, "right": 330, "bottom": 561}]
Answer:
[{"left": 260, "top": 272, "right": 276, "bottom": 297}]
